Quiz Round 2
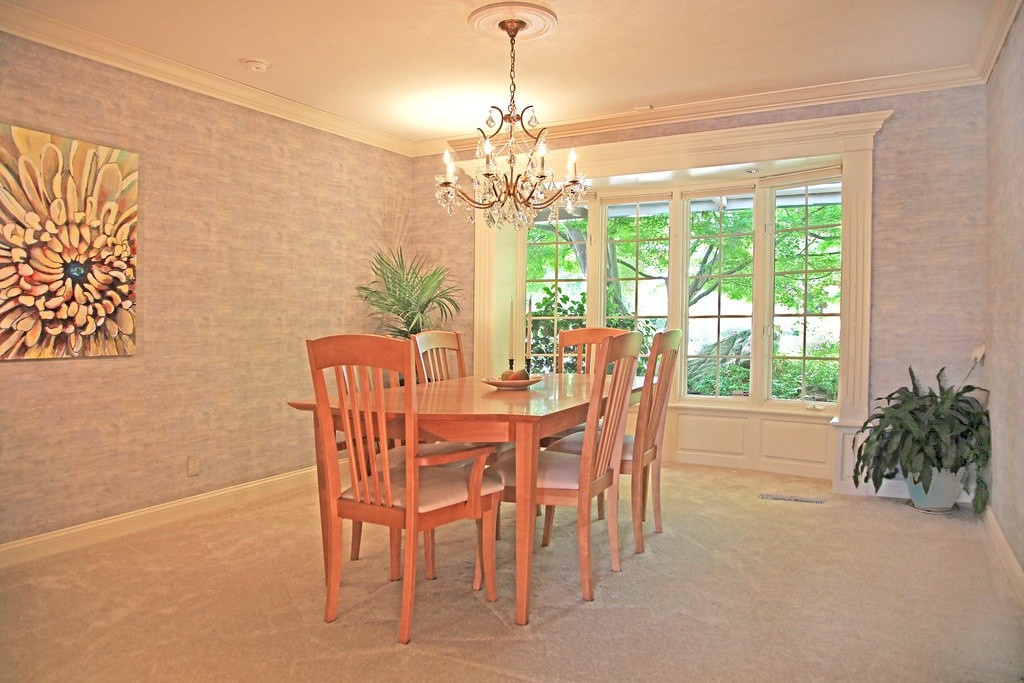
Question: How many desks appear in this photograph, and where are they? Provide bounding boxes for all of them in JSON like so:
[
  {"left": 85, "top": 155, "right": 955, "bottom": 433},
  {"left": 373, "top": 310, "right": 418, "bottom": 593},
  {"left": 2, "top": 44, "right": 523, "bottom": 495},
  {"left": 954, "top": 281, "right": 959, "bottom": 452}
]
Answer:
[{"left": 289, "top": 372, "right": 660, "bottom": 626}]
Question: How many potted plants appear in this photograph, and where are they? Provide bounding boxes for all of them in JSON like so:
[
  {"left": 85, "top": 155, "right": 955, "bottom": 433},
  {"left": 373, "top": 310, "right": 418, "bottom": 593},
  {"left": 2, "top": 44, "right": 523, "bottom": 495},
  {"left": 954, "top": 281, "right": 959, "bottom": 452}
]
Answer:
[
  {"left": 353, "top": 246, "right": 463, "bottom": 387},
  {"left": 851, "top": 367, "right": 994, "bottom": 516}
]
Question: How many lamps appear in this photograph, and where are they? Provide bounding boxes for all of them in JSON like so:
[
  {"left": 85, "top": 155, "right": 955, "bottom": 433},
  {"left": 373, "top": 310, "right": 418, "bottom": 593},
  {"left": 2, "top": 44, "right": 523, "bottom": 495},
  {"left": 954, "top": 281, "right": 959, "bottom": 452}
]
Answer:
[{"left": 435, "top": 2, "right": 586, "bottom": 231}]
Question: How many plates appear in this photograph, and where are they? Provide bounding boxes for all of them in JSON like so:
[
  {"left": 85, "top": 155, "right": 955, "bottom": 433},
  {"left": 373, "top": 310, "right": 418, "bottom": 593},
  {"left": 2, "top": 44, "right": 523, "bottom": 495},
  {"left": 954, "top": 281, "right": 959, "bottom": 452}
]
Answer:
[{"left": 482, "top": 376, "right": 544, "bottom": 390}]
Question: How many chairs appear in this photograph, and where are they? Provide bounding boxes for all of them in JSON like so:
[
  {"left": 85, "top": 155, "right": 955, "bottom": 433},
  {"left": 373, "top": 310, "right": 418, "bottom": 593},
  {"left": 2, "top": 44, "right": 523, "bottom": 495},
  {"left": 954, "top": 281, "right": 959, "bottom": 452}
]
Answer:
[
  {"left": 408, "top": 329, "right": 462, "bottom": 383},
  {"left": 471, "top": 329, "right": 643, "bottom": 627},
  {"left": 540, "top": 327, "right": 631, "bottom": 448},
  {"left": 541, "top": 327, "right": 686, "bottom": 556},
  {"left": 308, "top": 333, "right": 504, "bottom": 645}
]
